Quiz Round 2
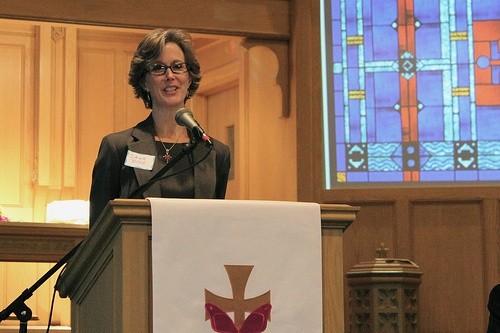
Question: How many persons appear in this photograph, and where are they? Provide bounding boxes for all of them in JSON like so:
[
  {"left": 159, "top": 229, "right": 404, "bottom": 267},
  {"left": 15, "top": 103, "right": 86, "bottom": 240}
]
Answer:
[{"left": 86, "top": 28, "right": 230, "bottom": 229}]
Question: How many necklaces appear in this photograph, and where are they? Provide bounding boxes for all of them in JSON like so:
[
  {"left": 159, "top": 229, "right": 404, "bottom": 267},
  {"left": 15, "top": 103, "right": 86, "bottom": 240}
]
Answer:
[{"left": 155, "top": 126, "right": 188, "bottom": 165}]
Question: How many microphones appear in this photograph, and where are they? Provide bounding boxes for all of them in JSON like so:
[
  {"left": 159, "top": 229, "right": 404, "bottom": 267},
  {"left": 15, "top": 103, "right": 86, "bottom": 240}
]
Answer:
[{"left": 175, "top": 107, "right": 213, "bottom": 147}]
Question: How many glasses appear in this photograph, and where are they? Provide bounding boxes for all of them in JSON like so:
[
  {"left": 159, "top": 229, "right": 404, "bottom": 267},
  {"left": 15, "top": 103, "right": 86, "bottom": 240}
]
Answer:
[{"left": 145, "top": 61, "right": 192, "bottom": 76}]
